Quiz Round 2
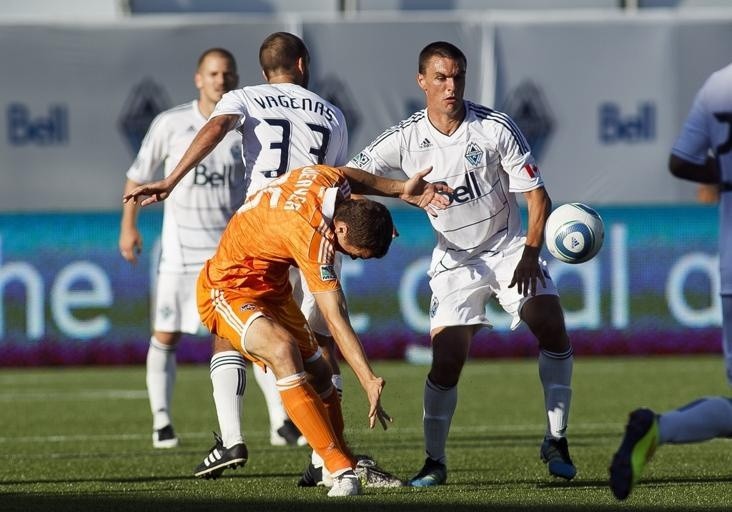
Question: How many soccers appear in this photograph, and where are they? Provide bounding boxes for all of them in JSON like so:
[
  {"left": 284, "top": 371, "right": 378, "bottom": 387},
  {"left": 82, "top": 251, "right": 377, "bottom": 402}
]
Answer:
[{"left": 543, "top": 202, "right": 604, "bottom": 263}]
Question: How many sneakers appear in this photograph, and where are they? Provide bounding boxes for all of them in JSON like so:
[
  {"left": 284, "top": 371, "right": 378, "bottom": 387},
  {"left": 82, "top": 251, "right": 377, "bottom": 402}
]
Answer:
[
  {"left": 607, "top": 407, "right": 660, "bottom": 502},
  {"left": 152, "top": 418, "right": 447, "bottom": 497},
  {"left": 539, "top": 431, "right": 577, "bottom": 480}
]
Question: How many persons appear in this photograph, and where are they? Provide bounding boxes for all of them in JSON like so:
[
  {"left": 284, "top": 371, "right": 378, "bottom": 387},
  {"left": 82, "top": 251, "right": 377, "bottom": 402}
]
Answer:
[
  {"left": 119, "top": 48, "right": 246, "bottom": 449},
  {"left": 121, "top": 32, "right": 348, "bottom": 479},
  {"left": 197, "top": 165, "right": 455, "bottom": 496},
  {"left": 344, "top": 41, "right": 576, "bottom": 488},
  {"left": 610, "top": 63, "right": 732, "bottom": 501}
]
